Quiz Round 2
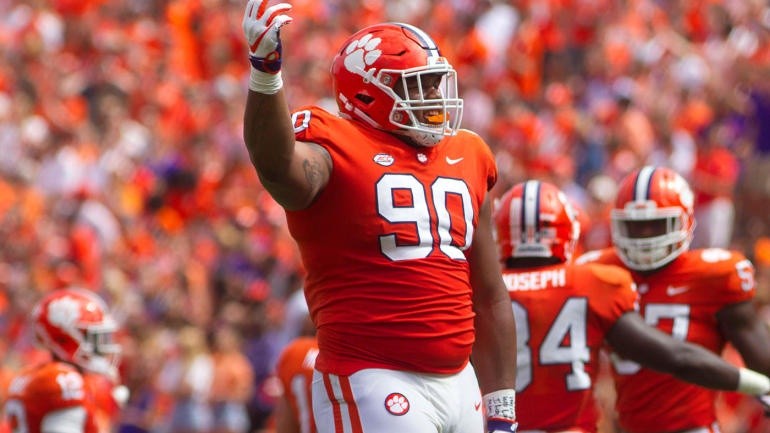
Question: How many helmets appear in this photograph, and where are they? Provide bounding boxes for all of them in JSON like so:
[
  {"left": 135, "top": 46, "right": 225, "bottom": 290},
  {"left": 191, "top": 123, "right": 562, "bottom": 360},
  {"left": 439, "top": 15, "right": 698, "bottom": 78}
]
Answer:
[
  {"left": 329, "top": 22, "right": 449, "bottom": 148},
  {"left": 609, "top": 167, "right": 696, "bottom": 270},
  {"left": 493, "top": 179, "right": 579, "bottom": 261},
  {"left": 34, "top": 289, "right": 119, "bottom": 378}
]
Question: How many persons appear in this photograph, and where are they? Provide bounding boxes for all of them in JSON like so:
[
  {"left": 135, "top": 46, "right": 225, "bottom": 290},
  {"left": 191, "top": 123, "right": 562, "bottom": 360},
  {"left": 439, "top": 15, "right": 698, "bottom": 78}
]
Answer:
[
  {"left": 576, "top": 164, "right": 770, "bottom": 433},
  {"left": 0, "top": 0, "right": 770, "bottom": 433},
  {"left": 492, "top": 182, "right": 770, "bottom": 433},
  {"left": 242, "top": 0, "right": 519, "bottom": 433},
  {"left": 0, "top": 288, "right": 131, "bottom": 431}
]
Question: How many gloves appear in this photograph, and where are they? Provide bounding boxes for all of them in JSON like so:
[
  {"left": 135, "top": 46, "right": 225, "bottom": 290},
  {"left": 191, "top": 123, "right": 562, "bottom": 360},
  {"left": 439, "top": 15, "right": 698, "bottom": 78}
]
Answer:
[
  {"left": 487, "top": 417, "right": 519, "bottom": 433},
  {"left": 243, "top": 0, "right": 292, "bottom": 95}
]
